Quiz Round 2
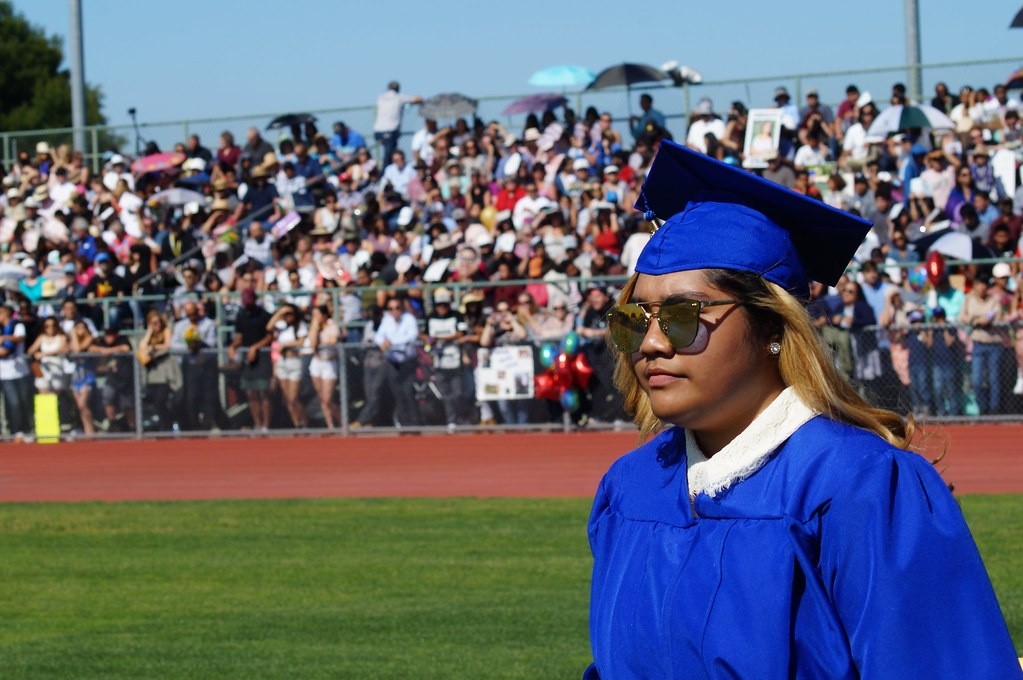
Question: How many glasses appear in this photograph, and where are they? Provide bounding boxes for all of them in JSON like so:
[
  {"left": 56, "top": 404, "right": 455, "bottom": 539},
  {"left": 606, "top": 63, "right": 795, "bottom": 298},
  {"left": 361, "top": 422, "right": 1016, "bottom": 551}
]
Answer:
[{"left": 607, "top": 297, "right": 743, "bottom": 353}]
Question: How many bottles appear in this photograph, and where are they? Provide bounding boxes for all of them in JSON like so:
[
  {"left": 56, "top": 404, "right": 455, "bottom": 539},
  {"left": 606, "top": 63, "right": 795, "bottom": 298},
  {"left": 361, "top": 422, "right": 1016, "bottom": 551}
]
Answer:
[{"left": 172, "top": 424, "right": 180, "bottom": 439}]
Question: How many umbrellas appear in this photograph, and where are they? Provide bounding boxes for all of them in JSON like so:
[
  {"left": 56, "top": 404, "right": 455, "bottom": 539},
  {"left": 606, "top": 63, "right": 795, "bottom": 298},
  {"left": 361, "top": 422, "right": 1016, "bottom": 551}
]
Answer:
[
  {"left": 931, "top": 233, "right": 995, "bottom": 265},
  {"left": 586, "top": 62, "right": 667, "bottom": 117},
  {"left": 131, "top": 152, "right": 184, "bottom": 173},
  {"left": 662, "top": 61, "right": 702, "bottom": 88},
  {"left": 417, "top": 93, "right": 478, "bottom": 127},
  {"left": 150, "top": 190, "right": 207, "bottom": 208},
  {"left": 869, "top": 101, "right": 957, "bottom": 134},
  {"left": 265, "top": 113, "right": 316, "bottom": 131},
  {"left": 528, "top": 64, "right": 597, "bottom": 96},
  {"left": 503, "top": 93, "right": 567, "bottom": 115}
]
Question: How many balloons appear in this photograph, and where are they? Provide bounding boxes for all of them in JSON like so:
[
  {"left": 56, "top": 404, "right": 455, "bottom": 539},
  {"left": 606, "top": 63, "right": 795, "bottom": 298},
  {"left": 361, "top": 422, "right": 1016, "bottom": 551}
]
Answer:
[
  {"left": 535, "top": 370, "right": 567, "bottom": 400},
  {"left": 553, "top": 354, "right": 576, "bottom": 389},
  {"left": 561, "top": 331, "right": 578, "bottom": 357},
  {"left": 559, "top": 391, "right": 579, "bottom": 411},
  {"left": 571, "top": 354, "right": 592, "bottom": 386},
  {"left": 538, "top": 343, "right": 558, "bottom": 368}
]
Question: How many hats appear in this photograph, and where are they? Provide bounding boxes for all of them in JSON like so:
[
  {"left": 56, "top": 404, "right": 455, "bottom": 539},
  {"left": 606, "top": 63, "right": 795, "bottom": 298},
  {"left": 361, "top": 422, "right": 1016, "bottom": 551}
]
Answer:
[{"left": 635, "top": 139, "right": 873, "bottom": 309}]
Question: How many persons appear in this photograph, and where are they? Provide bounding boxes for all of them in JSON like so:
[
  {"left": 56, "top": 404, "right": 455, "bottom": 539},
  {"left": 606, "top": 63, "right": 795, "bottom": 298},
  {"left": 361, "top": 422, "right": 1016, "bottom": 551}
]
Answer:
[
  {"left": 581, "top": 140, "right": 1023, "bottom": 680},
  {"left": 0, "top": 76, "right": 1023, "bottom": 439},
  {"left": 752, "top": 120, "right": 773, "bottom": 154}
]
